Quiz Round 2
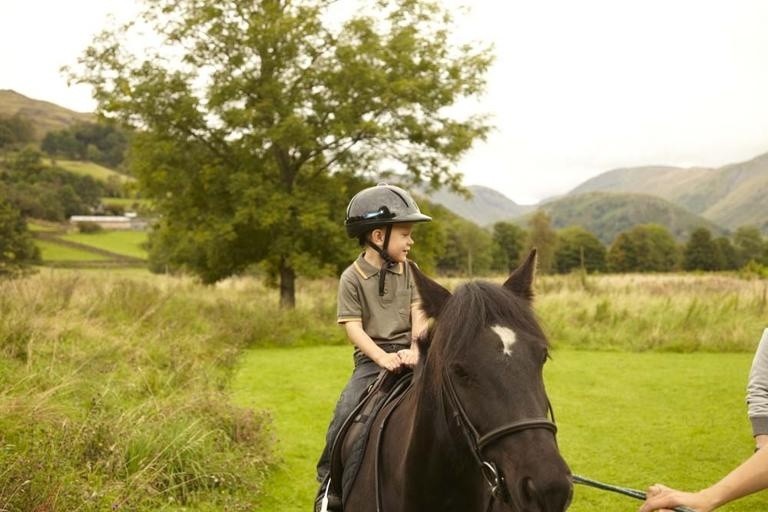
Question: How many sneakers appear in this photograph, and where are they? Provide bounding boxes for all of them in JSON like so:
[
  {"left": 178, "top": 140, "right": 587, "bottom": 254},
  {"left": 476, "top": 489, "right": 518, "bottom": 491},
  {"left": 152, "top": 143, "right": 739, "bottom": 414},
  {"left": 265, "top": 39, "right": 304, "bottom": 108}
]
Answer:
[{"left": 316, "top": 493, "right": 342, "bottom": 512}]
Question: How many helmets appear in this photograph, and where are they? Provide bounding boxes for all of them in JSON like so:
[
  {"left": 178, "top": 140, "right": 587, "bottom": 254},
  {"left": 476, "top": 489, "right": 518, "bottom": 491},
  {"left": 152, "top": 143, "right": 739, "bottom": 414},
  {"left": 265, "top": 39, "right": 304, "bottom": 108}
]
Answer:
[{"left": 344, "top": 182, "right": 433, "bottom": 239}]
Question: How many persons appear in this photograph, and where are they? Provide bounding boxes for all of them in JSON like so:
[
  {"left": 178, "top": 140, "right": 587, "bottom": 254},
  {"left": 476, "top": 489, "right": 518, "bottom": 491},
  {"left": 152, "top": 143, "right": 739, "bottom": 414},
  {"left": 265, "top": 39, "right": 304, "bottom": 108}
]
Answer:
[
  {"left": 311, "top": 183, "right": 436, "bottom": 511},
  {"left": 630, "top": 326, "right": 767, "bottom": 512}
]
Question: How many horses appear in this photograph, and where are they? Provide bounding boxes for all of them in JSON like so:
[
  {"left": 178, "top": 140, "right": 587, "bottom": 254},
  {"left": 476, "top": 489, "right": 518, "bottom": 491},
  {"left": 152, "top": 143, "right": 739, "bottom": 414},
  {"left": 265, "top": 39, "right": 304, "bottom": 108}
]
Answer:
[{"left": 330, "top": 248, "right": 575, "bottom": 512}]
